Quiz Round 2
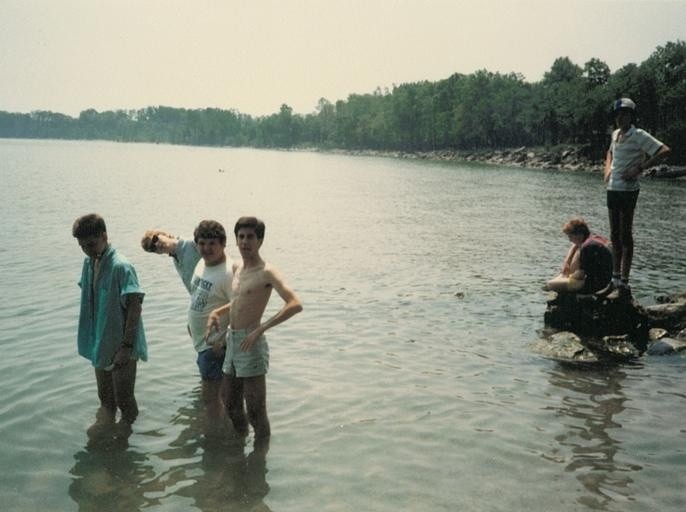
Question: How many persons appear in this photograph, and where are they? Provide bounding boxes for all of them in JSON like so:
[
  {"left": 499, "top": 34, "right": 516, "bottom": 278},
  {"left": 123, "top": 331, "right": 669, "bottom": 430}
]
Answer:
[
  {"left": 541, "top": 217, "right": 616, "bottom": 309},
  {"left": 69, "top": 212, "right": 148, "bottom": 421},
  {"left": 204, "top": 215, "right": 303, "bottom": 438},
  {"left": 601, "top": 96, "right": 673, "bottom": 302},
  {"left": 185, "top": 218, "right": 233, "bottom": 423},
  {"left": 140, "top": 230, "right": 203, "bottom": 295}
]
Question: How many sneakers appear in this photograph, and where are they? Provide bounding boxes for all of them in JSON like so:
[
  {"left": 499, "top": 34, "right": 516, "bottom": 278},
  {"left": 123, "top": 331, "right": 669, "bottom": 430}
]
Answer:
[{"left": 593, "top": 281, "right": 632, "bottom": 301}]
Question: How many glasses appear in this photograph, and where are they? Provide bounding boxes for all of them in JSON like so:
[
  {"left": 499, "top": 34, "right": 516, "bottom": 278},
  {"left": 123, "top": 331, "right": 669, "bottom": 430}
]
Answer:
[{"left": 149, "top": 232, "right": 161, "bottom": 252}]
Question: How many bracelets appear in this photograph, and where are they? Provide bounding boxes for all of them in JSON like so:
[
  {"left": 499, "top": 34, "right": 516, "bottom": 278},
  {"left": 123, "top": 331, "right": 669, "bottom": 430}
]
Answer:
[{"left": 637, "top": 165, "right": 644, "bottom": 172}]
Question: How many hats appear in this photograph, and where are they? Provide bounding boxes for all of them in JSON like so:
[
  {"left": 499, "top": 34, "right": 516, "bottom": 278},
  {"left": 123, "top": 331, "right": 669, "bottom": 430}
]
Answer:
[{"left": 614, "top": 98, "right": 636, "bottom": 113}]
